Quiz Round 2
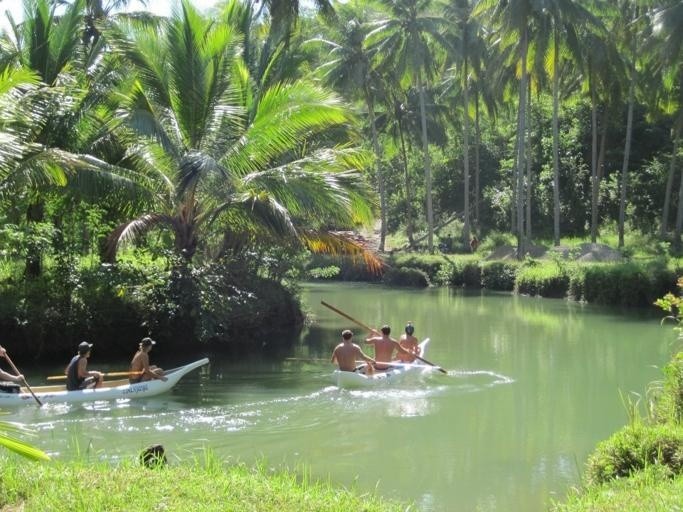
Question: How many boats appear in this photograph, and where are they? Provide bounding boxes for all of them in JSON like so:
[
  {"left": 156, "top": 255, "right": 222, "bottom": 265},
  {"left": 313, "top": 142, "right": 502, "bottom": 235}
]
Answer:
[
  {"left": 0, "top": 357, "right": 210, "bottom": 406},
  {"left": 332, "top": 337, "right": 431, "bottom": 389}
]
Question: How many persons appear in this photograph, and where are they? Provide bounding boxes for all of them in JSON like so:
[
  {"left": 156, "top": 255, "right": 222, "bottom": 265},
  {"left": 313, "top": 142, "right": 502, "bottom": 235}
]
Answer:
[
  {"left": 331, "top": 329, "right": 377, "bottom": 376},
  {"left": 396, "top": 322, "right": 421, "bottom": 362},
  {"left": 65, "top": 341, "right": 103, "bottom": 390},
  {"left": 0, "top": 347, "right": 24, "bottom": 394},
  {"left": 365, "top": 326, "right": 415, "bottom": 372},
  {"left": 129, "top": 337, "right": 168, "bottom": 383}
]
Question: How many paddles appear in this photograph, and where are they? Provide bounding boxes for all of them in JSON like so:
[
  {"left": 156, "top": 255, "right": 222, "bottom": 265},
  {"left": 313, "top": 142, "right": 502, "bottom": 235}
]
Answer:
[
  {"left": 46, "top": 371, "right": 142, "bottom": 380},
  {"left": 285, "top": 358, "right": 440, "bottom": 370},
  {"left": 320, "top": 301, "right": 446, "bottom": 374}
]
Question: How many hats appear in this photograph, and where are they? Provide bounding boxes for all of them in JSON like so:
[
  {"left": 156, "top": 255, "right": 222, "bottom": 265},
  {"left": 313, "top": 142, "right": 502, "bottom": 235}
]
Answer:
[
  {"left": 139, "top": 336, "right": 158, "bottom": 347},
  {"left": 78, "top": 340, "right": 94, "bottom": 350}
]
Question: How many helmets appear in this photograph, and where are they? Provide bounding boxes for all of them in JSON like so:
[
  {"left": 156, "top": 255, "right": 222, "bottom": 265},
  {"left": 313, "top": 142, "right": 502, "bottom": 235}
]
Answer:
[{"left": 405, "top": 323, "right": 415, "bottom": 335}]
